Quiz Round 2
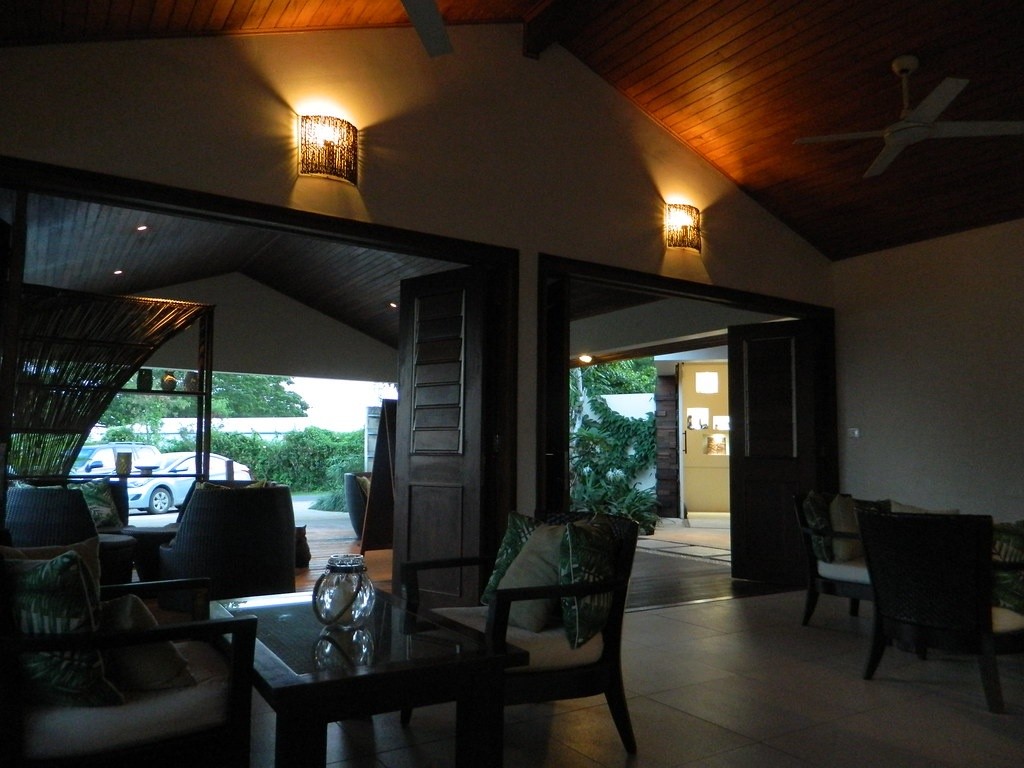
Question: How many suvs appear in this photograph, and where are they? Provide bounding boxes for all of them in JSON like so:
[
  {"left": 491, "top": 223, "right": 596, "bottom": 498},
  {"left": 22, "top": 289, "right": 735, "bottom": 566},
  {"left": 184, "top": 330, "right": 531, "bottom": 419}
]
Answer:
[{"left": 69, "top": 441, "right": 166, "bottom": 479}]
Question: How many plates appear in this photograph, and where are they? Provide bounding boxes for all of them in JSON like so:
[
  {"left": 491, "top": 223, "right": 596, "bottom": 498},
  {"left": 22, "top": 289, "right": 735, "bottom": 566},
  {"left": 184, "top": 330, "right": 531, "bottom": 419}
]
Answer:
[{"left": 135, "top": 465, "right": 160, "bottom": 475}]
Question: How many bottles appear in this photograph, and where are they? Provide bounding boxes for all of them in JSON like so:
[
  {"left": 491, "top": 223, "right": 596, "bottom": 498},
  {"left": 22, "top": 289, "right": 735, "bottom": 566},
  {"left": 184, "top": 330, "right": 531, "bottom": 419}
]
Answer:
[{"left": 314, "top": 553, "right": 376, "bottom": 628}]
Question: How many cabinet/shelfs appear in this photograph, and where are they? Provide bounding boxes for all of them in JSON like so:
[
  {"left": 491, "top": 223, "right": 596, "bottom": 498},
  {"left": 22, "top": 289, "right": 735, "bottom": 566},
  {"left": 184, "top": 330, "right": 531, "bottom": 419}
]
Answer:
[{"left": 10, "top": 282, "right": 219, "bottom": 478}]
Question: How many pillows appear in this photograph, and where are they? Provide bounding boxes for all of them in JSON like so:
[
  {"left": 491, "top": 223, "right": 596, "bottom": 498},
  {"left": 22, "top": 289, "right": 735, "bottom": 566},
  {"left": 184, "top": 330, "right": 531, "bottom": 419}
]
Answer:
[
  {"left": 804, "top": 489, "right": 891, "bottom": 564},
  {"left": 66, "top": 476, "right": 125, "bottom": 531},
  {"left": 5, "top": 551, "right": 126, "bottom": 707},
  {"left": 480, "top": 510, "right": 622, "bottom": 648}
]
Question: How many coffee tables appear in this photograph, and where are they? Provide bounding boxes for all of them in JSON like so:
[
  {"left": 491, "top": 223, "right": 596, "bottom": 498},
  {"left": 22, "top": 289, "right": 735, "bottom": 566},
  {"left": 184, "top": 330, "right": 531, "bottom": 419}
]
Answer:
[{"left": 210, "top": 586, "right": 529, "bottom": 768}]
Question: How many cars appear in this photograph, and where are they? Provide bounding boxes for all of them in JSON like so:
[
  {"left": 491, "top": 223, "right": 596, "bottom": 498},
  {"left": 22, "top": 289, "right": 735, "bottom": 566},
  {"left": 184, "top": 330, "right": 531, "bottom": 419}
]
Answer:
[{"left": 127, "top": 452, "right": 253, "bottom": 514}]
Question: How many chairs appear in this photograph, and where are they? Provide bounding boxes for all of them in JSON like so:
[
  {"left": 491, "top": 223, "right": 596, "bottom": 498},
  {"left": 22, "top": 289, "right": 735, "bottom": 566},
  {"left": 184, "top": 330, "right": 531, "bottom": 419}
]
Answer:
[
  {"left": 0, "top": 477, "right": 295, "bottom": 768},
  {"left": 399, "top": 510, "right": 640, "bottom": 757},
  {"left": 791, "top": 492, "right": 1024, "bottom": 714}
]
